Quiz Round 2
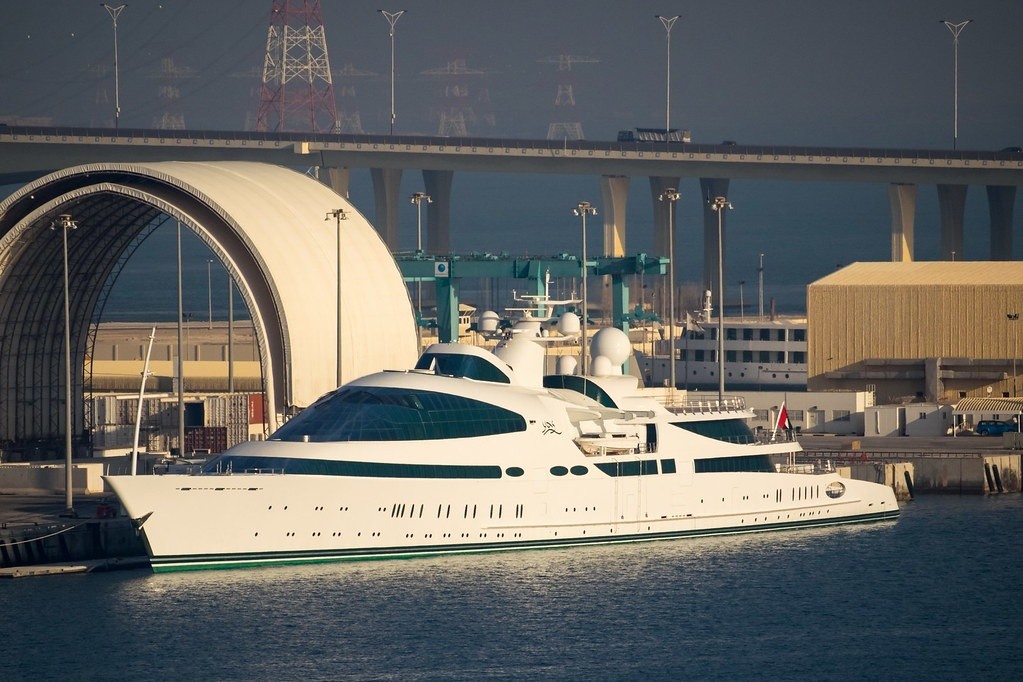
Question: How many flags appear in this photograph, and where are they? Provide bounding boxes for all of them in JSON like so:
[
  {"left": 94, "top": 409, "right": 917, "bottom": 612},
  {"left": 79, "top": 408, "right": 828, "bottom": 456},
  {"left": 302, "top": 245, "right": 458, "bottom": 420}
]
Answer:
[{"left": 778, "top": 406, "right": 795, "bottom": 441}]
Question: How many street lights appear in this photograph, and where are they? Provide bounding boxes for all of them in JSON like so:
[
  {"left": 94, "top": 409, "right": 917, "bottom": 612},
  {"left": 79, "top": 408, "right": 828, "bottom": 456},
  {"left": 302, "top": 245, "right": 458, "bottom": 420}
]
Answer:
[
  {"left": 205, "top": 259, "right": 213, "bottom": 329},
  {"left": 711, "top": 197, "right": 734, "bottom": 408},
  {"left": 658, "top": 187, "right": 681, "bottom": 386},
  {"left": 411, "top": 192, "right": 431, "bottom": 318},
  {"left": 51, "top": 216, "right": 78, "bottom": 516},
  {"left": 324, "top": 209, "right": 349, "bottom": 390},
  {"left": 183, "top": 313, "right": 192, "bottom": 361},
  {"left": 1007, "top": 312, "right": 1019, "bottom": 397},
  {"left": 572, "top": 202, "right": 598, "bottom": 376}
]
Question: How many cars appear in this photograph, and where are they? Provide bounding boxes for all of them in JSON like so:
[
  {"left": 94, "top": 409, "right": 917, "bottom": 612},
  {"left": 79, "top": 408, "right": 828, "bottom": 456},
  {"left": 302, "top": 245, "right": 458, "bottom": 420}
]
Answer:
[{"left": 976, "top": 420, "right": 1016, "bottom": 436}]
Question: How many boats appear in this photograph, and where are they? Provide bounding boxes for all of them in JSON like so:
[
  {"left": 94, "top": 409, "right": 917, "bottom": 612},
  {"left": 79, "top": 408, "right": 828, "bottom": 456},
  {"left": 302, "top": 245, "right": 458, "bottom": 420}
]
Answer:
[
  {"left": 101, "top": 345, "right": 901, "bottom": 575},
  {"left": 644, "top": 256, "right": 807, "bottom": 390}
]
